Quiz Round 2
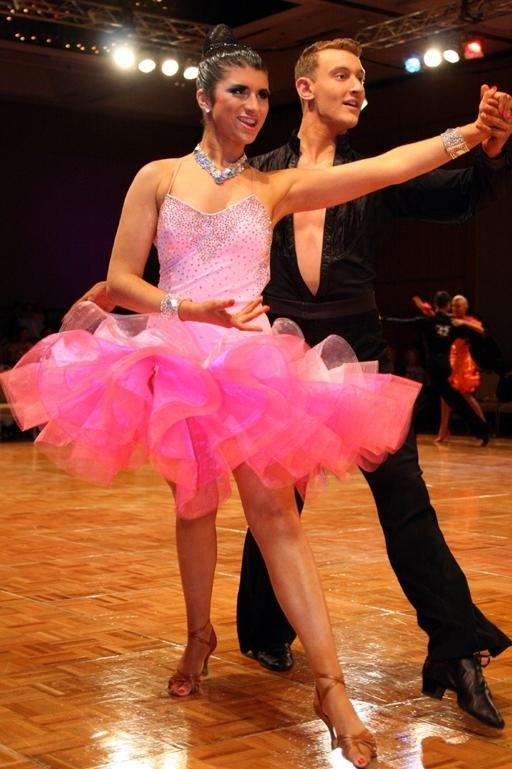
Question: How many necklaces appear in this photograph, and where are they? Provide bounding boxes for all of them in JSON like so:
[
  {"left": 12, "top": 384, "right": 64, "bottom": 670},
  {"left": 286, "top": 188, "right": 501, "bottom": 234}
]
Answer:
[{"left": 193, "top": 141, "right": 249, "bottom": 186}]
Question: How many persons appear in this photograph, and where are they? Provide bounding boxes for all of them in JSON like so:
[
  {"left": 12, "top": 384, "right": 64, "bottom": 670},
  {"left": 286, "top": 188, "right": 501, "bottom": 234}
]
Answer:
[
  {"left": 410, "top": 289, "right": 498, "bottom": 443},
  {"left": 1, "top": 37, "right": 509, "bottom": 769},
  {"left": 64, "top": 31, "right": 512, "bottom": 736},
  {"left": 372, "top": 288, "right": 484, "bottom": 434}
]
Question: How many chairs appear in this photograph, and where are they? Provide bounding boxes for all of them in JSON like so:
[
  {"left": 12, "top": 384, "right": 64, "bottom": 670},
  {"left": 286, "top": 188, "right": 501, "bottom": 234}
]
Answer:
[{"left": 472, "top": 369, "right": 512, "bottom": 438}]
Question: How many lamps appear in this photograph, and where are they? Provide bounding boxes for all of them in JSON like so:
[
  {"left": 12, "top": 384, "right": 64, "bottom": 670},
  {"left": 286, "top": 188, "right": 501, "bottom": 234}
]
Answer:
[
  {"left": 137, "top": 48, "right": 200, "bottom": 81},
  {"left": 401, "top": 30, "right": 463, "bottom": 74}
]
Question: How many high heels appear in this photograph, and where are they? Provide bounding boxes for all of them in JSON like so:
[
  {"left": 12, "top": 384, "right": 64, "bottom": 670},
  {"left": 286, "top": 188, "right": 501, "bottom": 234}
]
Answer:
[
  {"left": 168, "top": 620, "right": 217, "bottom": 696},
  {"left": 434, "top": 429, "right": 450, "bottom": 442},
  {"left": 257, "top": 643, "right": 294, "bottom": 671},
  {"left": 422, "top": 649, "right": 504, "bottom": 729},
  {"left": 314, "top": 674, "right": 378, "bottom": 765}
]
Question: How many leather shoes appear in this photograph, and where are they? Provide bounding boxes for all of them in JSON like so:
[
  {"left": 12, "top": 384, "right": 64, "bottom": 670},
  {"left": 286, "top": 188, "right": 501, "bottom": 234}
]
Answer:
[{"left": 481, "top": 427, "right": 496, "bottom": 446}]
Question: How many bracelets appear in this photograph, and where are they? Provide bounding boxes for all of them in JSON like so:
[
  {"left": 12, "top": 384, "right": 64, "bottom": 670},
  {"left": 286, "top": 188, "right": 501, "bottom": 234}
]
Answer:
[
  {"left": 158, "top": 292, "right": 193, "bottom": 323},
  {"left": 439, "top": 125, "right": 473, "bottom": 162}
]
